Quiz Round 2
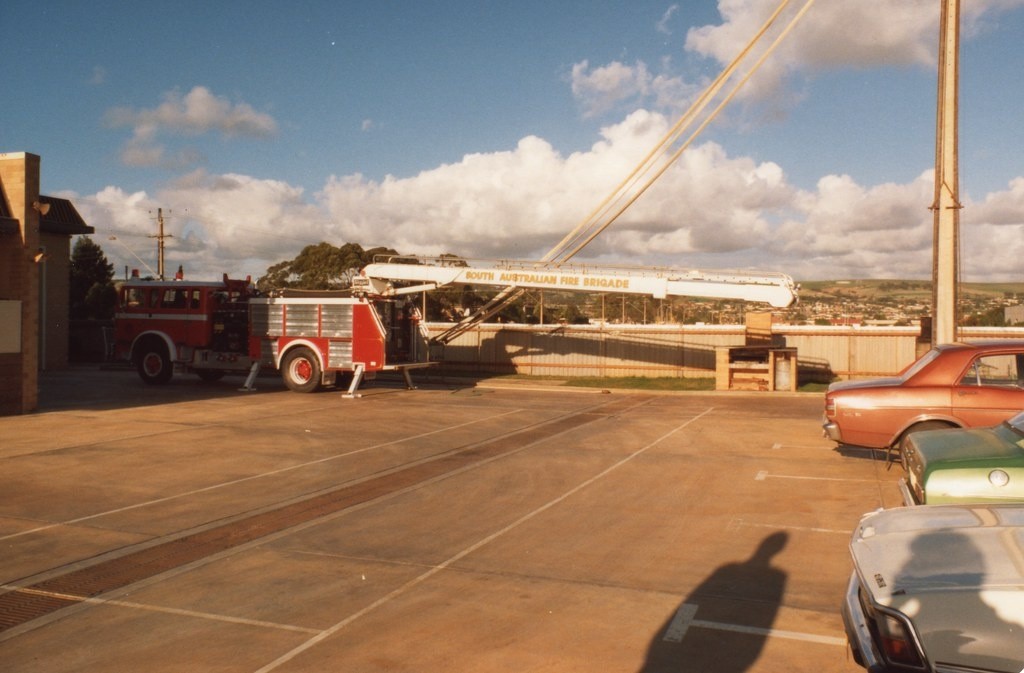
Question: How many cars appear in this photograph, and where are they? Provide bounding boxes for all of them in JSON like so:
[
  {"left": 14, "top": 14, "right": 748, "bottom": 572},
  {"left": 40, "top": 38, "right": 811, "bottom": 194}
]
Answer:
[
  {"left": 840, "top": 498, "right": 1024, "bottom": 673},
  {"left": 823, "top": 339, "right": 1024, "bottom": 470},
  {"left": 899, "top": 408, "right": 1024, "bottom": 510}
]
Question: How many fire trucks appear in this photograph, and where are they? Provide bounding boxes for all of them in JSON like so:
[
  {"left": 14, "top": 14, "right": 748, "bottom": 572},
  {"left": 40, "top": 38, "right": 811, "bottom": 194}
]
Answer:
[{"left": 111, "top": 264, "right": 798, "bottom": 401}]
